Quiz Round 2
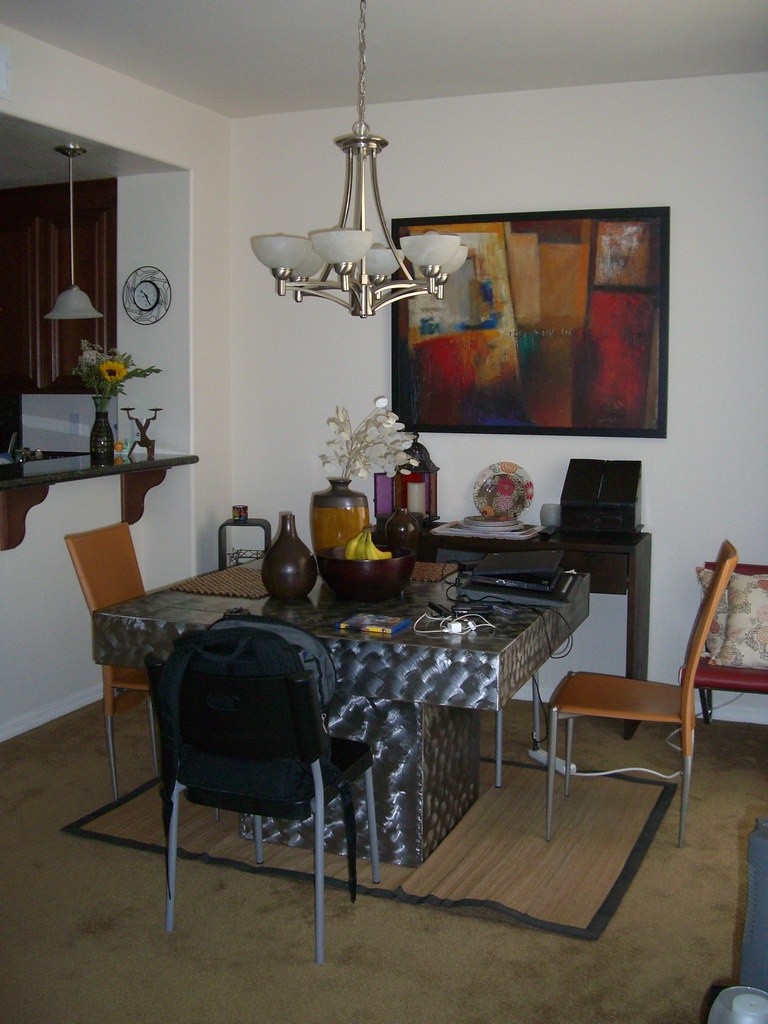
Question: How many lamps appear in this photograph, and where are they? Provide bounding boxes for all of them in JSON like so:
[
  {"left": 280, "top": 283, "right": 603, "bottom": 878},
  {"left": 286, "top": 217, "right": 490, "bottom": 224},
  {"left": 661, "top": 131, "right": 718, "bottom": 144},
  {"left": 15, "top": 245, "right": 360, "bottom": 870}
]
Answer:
[
  {"left": 250, "top": 0, "right": 469, "bottom": 318},
  {"left": 391, "top": 431, "right": 440, "bottom": 527},
  {"left": 44, "top": 144, "right": 104, "bottom": 319}
]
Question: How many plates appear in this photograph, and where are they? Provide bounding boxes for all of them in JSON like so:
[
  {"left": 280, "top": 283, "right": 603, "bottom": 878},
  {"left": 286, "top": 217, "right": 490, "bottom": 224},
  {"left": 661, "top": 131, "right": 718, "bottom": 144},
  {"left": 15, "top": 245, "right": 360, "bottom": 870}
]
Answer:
[{"left": 446, "top": 461, "right": 540, "bottom": 533}]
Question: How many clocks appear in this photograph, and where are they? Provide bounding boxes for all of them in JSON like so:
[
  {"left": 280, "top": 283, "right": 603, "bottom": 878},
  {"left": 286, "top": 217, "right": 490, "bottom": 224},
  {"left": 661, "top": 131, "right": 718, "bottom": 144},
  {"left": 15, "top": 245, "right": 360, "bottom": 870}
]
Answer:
[{"left": 122, "top": 266, "right": 171, "bottom": 325}]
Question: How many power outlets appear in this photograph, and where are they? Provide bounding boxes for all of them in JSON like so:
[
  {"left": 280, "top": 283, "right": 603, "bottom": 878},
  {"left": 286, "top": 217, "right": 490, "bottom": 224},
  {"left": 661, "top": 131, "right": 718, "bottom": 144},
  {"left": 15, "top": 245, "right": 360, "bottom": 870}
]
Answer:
[
  {"left": 70, "top": 414, "right": 80, "bottom": 435},
  {"left": 131, "top": 420, "right": 145, "bottom": 442}
]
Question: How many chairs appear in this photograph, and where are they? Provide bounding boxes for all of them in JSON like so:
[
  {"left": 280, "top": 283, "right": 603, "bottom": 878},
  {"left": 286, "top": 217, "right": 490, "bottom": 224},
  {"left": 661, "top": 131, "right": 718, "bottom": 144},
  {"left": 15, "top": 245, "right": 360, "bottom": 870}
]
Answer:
[
  {"left": 546, "top": 540, "right": 738, "bottom": 848},
  {"left": 164, "top": 626, "right": 380, "bottom": 965},
  {"left": 64, "top": 521, "right": 160, "bottom": 799},
  {"left": 678, "top": 561, "right": 768, "bottom": 724}
]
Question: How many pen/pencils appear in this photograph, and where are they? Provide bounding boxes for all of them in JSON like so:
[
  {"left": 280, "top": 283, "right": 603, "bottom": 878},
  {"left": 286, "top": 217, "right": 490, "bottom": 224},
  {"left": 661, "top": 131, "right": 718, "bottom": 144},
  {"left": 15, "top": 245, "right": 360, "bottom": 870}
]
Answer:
[{"left": 487, "top": 605, "right": 517, "bottom": 617}]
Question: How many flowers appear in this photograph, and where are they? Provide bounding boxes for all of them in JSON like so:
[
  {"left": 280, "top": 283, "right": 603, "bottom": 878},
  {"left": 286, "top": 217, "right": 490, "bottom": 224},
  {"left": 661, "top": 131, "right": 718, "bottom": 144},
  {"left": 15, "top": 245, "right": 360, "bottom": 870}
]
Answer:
[{"left": 76, "top": 339, "right": 162, "bottom": 398}]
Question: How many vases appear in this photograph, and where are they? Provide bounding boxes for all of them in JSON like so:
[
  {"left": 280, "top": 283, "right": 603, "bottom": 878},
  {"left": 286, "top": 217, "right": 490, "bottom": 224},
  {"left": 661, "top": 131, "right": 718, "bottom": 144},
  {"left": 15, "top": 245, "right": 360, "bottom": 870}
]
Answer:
[
  {"left": 90, "top": 396, "right": 114, "bottom": 467},
  {"left": 310, "top": 479, "right": 369, "bottom": 554},
  {"left": 261, "top": 514, "right": 317, "bottom": 600}
]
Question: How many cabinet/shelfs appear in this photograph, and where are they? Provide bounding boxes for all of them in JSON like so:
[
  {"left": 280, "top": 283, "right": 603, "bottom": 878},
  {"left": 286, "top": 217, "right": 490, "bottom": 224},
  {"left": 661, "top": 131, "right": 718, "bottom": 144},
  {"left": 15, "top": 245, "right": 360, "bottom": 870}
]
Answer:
[{"left": 0, "top": 178, "right": 117, "bottom": 394}]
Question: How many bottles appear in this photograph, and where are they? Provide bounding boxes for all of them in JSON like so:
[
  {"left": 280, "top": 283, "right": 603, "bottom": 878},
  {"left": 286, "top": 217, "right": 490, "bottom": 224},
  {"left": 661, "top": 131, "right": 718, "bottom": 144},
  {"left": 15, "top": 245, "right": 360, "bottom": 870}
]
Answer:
[
  {"left": 385, "top": 506, "right": 420, "bottom": 548},
  {"left": 0, "top": 448, "right": 43, "bottom": 465},
  {"left": 262, "top": 514, "right": 316, "bottom": 600}
]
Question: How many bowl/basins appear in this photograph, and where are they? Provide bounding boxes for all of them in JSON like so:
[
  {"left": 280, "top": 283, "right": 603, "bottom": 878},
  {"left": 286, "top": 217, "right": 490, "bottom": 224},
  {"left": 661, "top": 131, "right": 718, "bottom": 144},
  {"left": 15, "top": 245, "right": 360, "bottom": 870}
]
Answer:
[{"left": 318, "top": 545, "right": 417, "bottom": 601}]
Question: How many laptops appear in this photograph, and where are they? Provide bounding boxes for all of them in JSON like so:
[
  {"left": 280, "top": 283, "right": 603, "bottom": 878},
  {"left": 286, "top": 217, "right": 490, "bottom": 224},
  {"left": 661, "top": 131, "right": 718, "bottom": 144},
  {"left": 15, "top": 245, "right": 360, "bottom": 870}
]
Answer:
[{"left": 472, "top": 549, "right": 564, "bottom": 591}]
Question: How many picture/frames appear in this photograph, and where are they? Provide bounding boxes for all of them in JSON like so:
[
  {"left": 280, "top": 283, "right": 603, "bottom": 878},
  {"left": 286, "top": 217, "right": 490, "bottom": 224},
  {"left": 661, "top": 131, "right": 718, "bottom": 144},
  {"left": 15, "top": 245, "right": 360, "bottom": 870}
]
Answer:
[{"left": 391, "top": 207, "right": 670, "bottom": 438}]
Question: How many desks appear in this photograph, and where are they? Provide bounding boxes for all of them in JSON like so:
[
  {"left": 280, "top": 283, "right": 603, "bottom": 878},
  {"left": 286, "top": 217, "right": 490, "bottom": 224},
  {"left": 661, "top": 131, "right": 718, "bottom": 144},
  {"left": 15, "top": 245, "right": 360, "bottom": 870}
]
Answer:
[
  {"left": 371, "top": 523, "right": 652, "bottom": 740},
  {"left": 219, "top": 518, "right": 271, "bottom": 571},
  {"left": 91, "top": 558, "right": 590, "bottom": 869}
]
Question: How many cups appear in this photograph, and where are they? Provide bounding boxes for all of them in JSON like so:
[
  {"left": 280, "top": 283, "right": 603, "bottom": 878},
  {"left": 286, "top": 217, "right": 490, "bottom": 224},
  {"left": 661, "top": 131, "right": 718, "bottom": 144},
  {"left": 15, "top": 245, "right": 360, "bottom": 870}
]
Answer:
[
  {"left": 540, "top": 503, "right": 561, "bottom": 528},
  {"left": 233, "top": 504, "right": 247, "bottom": 522}
]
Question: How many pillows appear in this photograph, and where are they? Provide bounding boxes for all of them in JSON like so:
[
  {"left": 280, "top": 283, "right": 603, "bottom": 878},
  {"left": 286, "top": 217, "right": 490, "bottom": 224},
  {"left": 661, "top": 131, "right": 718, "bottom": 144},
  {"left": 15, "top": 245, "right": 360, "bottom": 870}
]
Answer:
[{"left": 696, "top": 566, "right": 768, "bottom": 670}]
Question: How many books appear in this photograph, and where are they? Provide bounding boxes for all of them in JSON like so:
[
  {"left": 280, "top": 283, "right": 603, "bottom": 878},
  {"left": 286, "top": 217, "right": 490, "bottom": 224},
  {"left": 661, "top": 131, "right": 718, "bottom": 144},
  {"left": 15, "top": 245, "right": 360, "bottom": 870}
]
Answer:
[{"left": 336, "top": 612, "right": 412, "bottom": 633}]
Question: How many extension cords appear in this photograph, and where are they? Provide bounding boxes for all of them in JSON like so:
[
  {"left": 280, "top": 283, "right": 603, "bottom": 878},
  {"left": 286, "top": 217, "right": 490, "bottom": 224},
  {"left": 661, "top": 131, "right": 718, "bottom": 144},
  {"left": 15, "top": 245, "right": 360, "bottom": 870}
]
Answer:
[{"left": 529, "top": 748, "right": 576, "bottom": 774}]
